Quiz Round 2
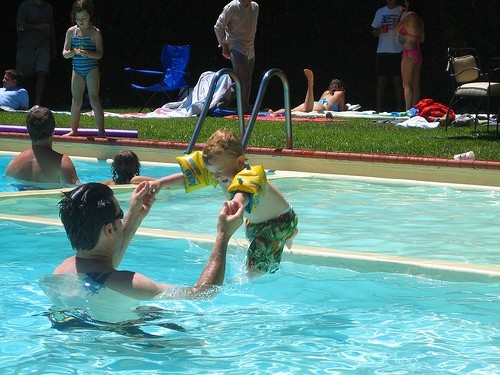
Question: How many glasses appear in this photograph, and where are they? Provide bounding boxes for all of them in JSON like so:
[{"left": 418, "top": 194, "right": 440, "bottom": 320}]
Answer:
[{"left": 99, "top": 210, "right": 124, "bottom": 228}]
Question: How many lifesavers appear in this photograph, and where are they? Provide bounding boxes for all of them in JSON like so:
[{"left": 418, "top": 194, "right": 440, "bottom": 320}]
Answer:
[
  {"left": 227, "top": 165, "right": 268, "bottom": 214},
  {"left": 176, "top": 151, "right": 218, "bottom": 194}
]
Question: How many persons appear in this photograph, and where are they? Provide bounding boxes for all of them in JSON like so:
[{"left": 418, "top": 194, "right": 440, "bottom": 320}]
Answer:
[
  {"left": 214, "top": 0, "right": 260, "bottom": 115},
  {"left": 62, "top": 0, "right": 106, "bottom": 138},
  {"left": 16, "top": 0, "right": 57, "bottom": 103},
  {"left": 268, "top": 68, "right": 346, "bottom": 118},
  {"left": 0, "top": 68, "right": 29, "bottom": 112},
  {"left": 100, "top": 149, "right": 157, "bottom": 185},
  {"left": 51, "top": 181, "right": 245, "bottom": 302},
  {"left": 369, "top": 0, "right": 407, "bottom": 112},
  {"left": 148, "top": 128, "right": 299, "bottom": 278},
  {"left": 4, "top": 106, "right": 79, "bottom": 184},
  {"left": 392, "top": 0, "right": 425, "bottom": 111}
]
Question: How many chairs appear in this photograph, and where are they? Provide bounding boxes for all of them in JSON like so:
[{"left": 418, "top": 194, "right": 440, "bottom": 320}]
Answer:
[
  {"left": 124, "top": 44, "right": 191, "bottom": 113},
  {"left": 446, "top": 46, "right": 500, "bottom": 132}
]
